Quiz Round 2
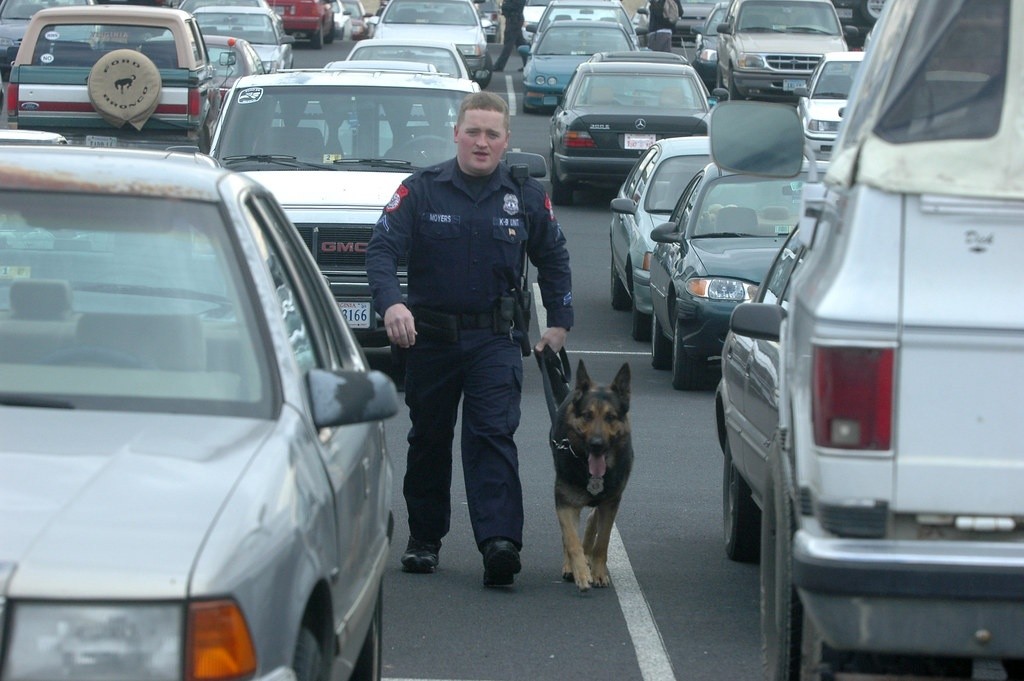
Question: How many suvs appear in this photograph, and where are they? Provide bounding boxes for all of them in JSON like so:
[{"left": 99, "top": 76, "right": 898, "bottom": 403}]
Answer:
[{"left": 165, "top": 70, "right": 547, "bottom": 360}]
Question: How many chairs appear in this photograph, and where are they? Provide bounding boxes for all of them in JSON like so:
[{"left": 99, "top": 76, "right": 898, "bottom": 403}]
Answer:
[
  {"left": 75, "top": 312, "right": 208, "bottom": 376},
  {"left": 716, "top": 207, "right": 759, "bottom": 234},
  {"left": 255, "top": 125, "right": 326, "bottom": 165}
]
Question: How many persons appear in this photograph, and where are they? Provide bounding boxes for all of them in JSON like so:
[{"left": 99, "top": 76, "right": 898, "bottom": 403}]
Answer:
[
  {"left": 492, "top": 0, "right": 531, "bottom": 72},
  {"left": 648, "top": 0, "right": 684, "bottom": 34},
  {"left": 366, "top": 91, "right": 575, "bottom": 586}
]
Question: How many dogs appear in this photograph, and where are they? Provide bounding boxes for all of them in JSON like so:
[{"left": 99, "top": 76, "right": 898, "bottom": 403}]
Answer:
[{"left": 544, "top": 359, "right": 635, "bottom": 589}]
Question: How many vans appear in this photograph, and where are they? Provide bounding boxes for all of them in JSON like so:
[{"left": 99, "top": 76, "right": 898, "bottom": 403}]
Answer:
[{"left": 708, "top": 1, "right": 1024, "bottom": 681}]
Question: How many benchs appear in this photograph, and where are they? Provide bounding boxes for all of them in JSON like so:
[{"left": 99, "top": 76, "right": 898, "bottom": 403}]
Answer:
[{"left": 0, "top": 279, "right": 243, "bottom": 371}]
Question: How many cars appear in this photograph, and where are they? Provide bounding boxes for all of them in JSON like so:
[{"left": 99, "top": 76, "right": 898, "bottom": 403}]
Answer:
[
  {"left": 0, "top": 0, "right": 375, "bottom": 152},
  {"left": 606, "top": 137, "right": 715, "bottom": 337},
  {"left": 717, "top": 0, "right": 887, "bottom": 101},
  {"left": 792, "top": 53, "right": 866, "bottom": 158},
  {"left": 545, "top": 64, "right": 730, "bottom": 207},
  {"left": 714, "top": 215, "right": 807, "bottom": 561},
  {"left": 295, "top": 0, "right": 500, "bottom": 149},
  {"left": 649, "top": 160, "right": 835, "bottom": 392},
  {"left": 0, "top": 135, "right": 401, "bottom": 681},
  {"left": 517, "top": 0, "right": 730, "bottom": 104}
]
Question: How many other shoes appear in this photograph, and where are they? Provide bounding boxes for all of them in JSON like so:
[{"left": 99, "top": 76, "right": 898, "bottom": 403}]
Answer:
[
  {"left": 400, "top": 538, "right": 442, "bottom": 572},
  {"left": 483, "top": 540, "right": 522, "bottom": 586}
]
